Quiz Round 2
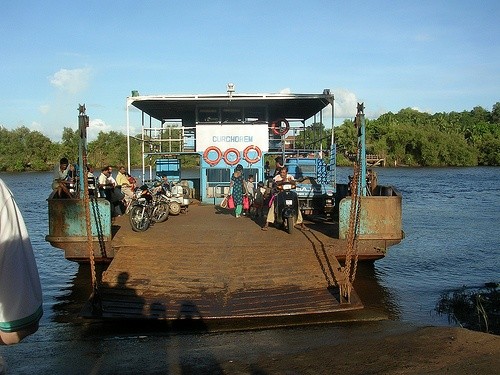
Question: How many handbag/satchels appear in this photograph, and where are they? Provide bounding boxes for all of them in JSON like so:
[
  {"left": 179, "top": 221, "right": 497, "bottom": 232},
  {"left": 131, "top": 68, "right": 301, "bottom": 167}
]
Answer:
[{"left": 220, "top": 194, "right": 235, "bottom": 209}]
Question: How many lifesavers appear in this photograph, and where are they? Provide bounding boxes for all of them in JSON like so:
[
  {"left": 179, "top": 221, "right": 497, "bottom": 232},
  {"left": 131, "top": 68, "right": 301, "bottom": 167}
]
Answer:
[
  {"left": 223, "top": 146, "right": 240, "bottom": 165},
  {"left": 242, "top": 145, "right": 263, "bottom": 164},
  {"left": 202, "top": 146, "right": 221, "bottom": 165},
  {"left": 270, "top": 117, "right": 290, "bottom": 134}
]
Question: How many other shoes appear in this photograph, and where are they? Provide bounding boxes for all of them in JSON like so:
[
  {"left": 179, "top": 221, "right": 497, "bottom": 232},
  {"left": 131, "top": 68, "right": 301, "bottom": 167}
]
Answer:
[
  {"left": 300, "top": 226, "right": 311, "bottom": 231},
  {"left": 262, "top": 227, "right": 268, "bottom": 231},
  {"left": 236, "top": 215, "right": 240, "bottom": 219}
]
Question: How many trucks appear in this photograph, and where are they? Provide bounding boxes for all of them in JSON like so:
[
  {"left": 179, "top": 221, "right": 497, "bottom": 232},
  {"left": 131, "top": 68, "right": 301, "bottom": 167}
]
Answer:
[{"left": 282, "top": 158, "right": 337, "bottom": 218}]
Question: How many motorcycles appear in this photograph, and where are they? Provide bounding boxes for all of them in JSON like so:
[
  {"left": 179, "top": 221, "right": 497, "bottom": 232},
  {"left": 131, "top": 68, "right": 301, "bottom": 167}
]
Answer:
[
  {"left": 268, "top": 176, "right": 306, "bottom": 233},
  {"left": 127, "top": 179, "right": 192, "bottom": 232}
]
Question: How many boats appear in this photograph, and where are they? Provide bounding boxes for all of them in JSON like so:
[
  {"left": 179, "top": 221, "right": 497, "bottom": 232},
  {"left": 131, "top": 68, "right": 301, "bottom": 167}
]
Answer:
[{"left": 43, "top": 82, "right": 403, "bottom": 322}]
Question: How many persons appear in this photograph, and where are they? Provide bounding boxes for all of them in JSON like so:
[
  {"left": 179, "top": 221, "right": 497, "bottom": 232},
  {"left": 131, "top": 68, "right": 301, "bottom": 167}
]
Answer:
[
  {"left": 228, "top": 152, "right": 310, "bottom": 231},
  {"left": 0, "top": 178, "right": 43, "bottom": 345},
  {"left": 52, "top": 158, "right": 170, "bottom": 217},
  {"left": 366, "top": 169, "right": 378, "bottom": 195}
]
